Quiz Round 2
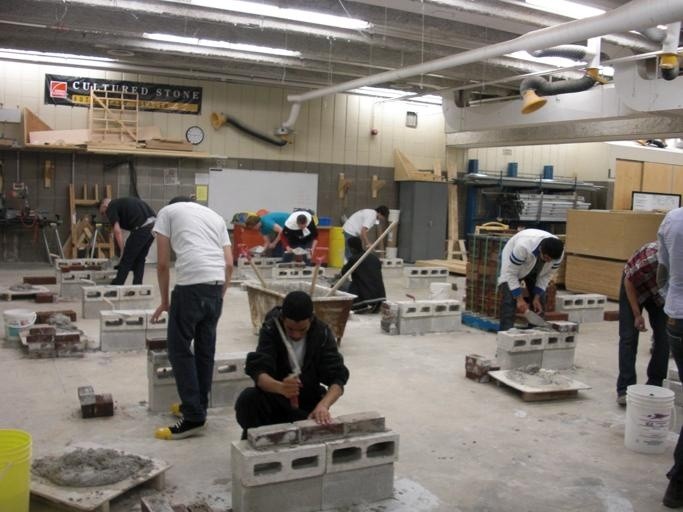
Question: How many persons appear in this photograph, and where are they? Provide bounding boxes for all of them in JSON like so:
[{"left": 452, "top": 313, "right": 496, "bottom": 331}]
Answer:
[
  {"left": 616, "top": 241, "right": 670, "bottom": 405},
  {"left": 94, "top": 197, "right": 159, "bottom": 287},
  {"left": 246, "top": 210, "right": 292, "bottom": 262},
  {"left": 150, "top": 192, "right": 234, "bottom": 442},
  {"left": 497, "top": 228, "right": 564, "bottom": 331},
  {"left": 334, "top": 237, "right": 386, "bottom": 315},
  {"left": 233, "top": 290, "right": 348, "bottom": 445},
  {"left": 341, "top": 204, "right": 390, "bottom": 264},
  {"left": 648, "top": 208, "right": 682, "bottom": 511},
  {"left": 281, "top": 210, "right": 320, "bottom": 267}
]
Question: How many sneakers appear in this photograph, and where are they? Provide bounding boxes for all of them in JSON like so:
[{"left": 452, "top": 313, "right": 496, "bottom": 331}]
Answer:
[{"left": 153, "top": 403, "right": 206, "bottom": 440}]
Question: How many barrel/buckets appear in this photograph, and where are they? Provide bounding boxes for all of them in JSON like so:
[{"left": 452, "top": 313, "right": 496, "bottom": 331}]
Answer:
[
  {"left": 0, "top": 428, "right": 32, "bottom": 512},
  {"left": 386, "top": 247, "right": 397, "bottom": 258},
  {"left": 625, "top": 383, "right": 676, "bottom": 455},
  {"left": 328, "top": 226, "right": 345, "bottom": 268},
  {"left": 430, "top": 282, "right": 452, "bottom": 300},
  {"left": 387, "top": 209, "right": 401, "bottom": 222},
  {"left": 4, "top": 308, "right": 37, "bottom": 341}
]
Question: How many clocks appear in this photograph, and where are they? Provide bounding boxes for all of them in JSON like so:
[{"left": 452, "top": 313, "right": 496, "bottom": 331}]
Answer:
[{"left": 185, "top": 126, "right": 204, "bottom": 145}]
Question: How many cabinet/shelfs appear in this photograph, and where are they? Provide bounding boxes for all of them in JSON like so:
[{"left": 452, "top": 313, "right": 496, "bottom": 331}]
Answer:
[
  {"left": 395, "top": 182, "right": 447, "bottom": 264},
  {"left": 466, "top": 176, "right": 613, "bottom": 238}
]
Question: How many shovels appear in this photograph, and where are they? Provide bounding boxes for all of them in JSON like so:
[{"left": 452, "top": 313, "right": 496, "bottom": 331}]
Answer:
[{"left": 523, "top": 307, "right": 549, "bottom": 327}]
